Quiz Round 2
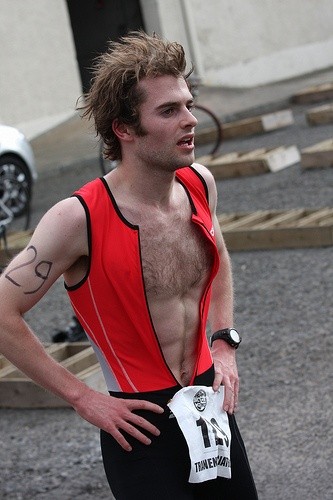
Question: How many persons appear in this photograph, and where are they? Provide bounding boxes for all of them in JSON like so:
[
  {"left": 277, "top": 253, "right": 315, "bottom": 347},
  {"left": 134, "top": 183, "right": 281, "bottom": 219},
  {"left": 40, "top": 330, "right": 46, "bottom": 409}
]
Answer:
[{"left": 1, "top": 29, "right": 258, "bottom": 500}]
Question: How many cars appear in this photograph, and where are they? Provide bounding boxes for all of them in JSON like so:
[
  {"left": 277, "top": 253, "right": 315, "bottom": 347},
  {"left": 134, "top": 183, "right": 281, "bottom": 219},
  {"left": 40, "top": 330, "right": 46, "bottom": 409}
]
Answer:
[{"left": 0, "top": 124, "right": 37, "bottom": 229}]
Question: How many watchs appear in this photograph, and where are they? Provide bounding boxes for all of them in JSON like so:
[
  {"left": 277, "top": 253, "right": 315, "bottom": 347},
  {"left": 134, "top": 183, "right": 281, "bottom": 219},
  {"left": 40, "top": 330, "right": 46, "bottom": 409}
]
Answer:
[{"left": 210, "top": 328, "right": 242, "bottom": 350}]
